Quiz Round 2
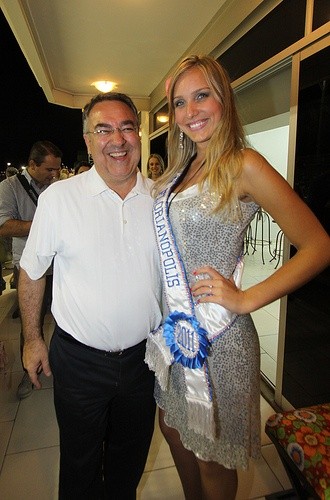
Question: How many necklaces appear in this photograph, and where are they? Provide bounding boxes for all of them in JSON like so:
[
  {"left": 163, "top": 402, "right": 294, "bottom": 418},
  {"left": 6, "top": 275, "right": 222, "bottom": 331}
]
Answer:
[{"left": 167, "top": 158, "right": 207, "bottom": 213}]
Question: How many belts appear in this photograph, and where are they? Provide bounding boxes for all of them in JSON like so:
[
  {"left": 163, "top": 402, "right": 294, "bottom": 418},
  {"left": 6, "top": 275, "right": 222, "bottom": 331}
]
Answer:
[{"left": 57, "top": 331, "right": 147, "bottom": 357}]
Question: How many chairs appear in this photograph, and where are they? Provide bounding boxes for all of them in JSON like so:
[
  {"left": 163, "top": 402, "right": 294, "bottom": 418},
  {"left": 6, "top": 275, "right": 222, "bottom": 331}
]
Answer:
[{"left": 264, "top": 405, "right": 330, "bottom": 500}]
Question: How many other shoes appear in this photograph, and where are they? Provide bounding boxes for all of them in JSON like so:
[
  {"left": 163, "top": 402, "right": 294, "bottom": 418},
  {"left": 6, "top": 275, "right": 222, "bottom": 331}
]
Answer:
[{"left": 17, "top": 372, "right": 39, "bottom": 398}]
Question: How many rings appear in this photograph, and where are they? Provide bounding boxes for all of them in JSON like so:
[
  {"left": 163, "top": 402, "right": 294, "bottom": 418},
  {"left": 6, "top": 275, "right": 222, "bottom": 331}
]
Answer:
[{"left": 208, "top": 286, "right": 212, "bottom": 296}]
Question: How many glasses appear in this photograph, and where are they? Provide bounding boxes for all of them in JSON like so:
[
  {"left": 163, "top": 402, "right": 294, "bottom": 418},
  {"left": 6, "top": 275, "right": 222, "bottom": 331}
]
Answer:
[{"left": 87, "top": 126, "right": 139, "bottom": 136}]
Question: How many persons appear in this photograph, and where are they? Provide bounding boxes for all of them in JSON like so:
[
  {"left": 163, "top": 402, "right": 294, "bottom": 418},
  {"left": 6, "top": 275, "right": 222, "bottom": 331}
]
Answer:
[
  {"left": 145, "top": 55, "right": 330, "bottom": 500},
  {"left": 0, "top": 142, "right": 62, "bottom": 400},
  {"left": 147, "top": 153, "right": 165, "bottom": 180},
  {"left": 18, "top": 93, "right": 167, "bottom": 500},
  {"left": 0, "top": 161, "right": 92, "bottom": 320}
]
